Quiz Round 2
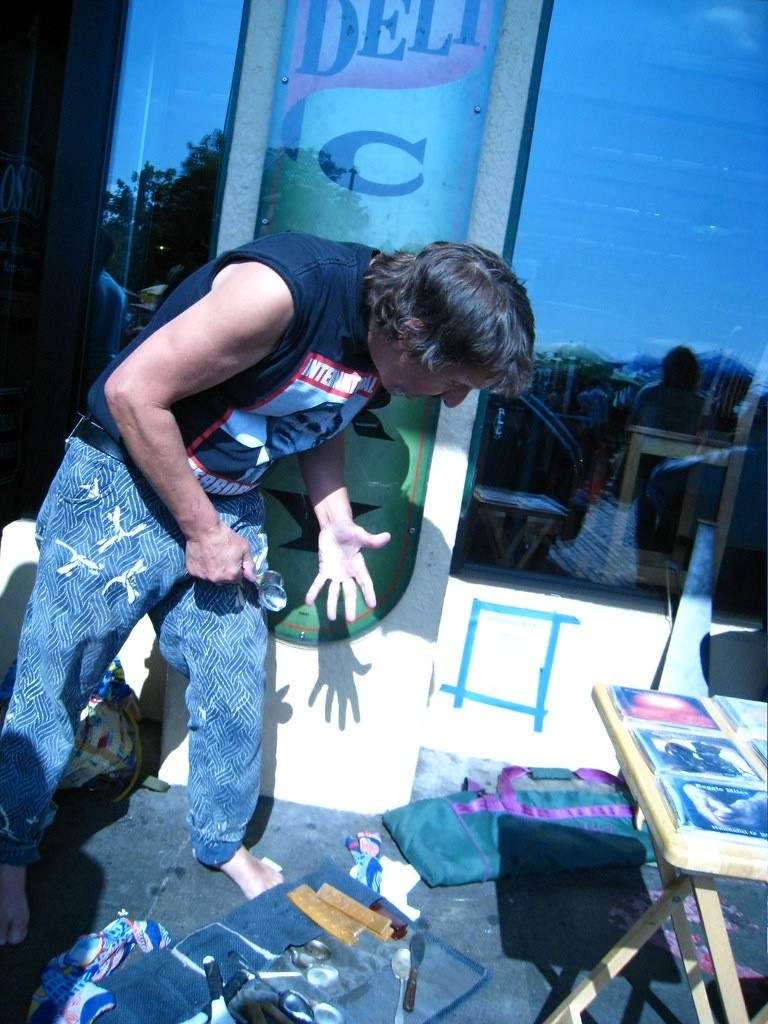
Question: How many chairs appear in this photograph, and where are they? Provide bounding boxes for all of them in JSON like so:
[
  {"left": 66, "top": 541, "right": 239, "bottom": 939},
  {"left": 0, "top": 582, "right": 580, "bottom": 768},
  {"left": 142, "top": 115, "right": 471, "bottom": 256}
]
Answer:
[{"left": 603, "top": 326, "right": 768, "bottom": 598}]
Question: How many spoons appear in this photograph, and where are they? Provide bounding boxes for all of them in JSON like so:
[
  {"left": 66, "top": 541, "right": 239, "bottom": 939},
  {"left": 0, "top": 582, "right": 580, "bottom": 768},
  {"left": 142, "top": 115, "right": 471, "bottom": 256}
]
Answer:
[
  {"left": 229, "top": 951, "right": 314, "bottom": 1024},
  {"left": 304, "top": 940, "right": 332, "bottom": 960},
  {"left": 243, "top": 964, "right": 339, "bottom": 986},
  {"left": 298, "top": 988, "right": 346, "bottom": 1024},
  {"left": 392, "top": 949, "right": 413, "bottom": 1024}
]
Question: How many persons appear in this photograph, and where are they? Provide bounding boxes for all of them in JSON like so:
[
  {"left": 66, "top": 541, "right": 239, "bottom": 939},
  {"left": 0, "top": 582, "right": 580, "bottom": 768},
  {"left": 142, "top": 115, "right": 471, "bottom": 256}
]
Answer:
[
  {"left": 0, "top": 232, "right": 536, "bottom": 945},
  {"left": 625, "top": 345, "right": 705, "bottom": 587}
]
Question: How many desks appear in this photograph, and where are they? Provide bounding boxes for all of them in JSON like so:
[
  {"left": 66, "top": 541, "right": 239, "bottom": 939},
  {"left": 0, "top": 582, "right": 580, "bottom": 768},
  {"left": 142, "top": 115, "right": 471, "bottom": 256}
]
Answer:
[
  {"left": 473, "top": 484, "right": 570, "bottom": 569},
  {"left": 542, "top": 683, "right": 768, "bottom": 1024}
]
